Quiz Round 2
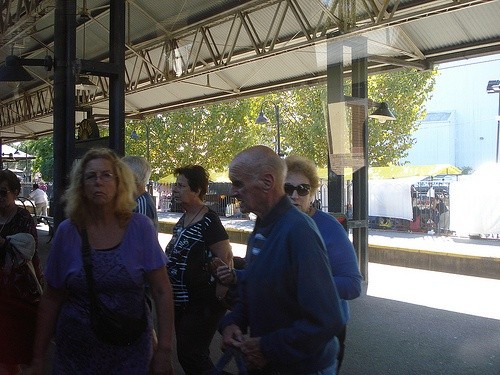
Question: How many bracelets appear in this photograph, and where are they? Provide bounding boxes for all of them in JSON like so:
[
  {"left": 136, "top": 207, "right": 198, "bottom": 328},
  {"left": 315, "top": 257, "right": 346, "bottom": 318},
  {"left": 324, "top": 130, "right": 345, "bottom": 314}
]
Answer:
[{"left": 217, "top": 296, "right": 223, "bottom": 300}]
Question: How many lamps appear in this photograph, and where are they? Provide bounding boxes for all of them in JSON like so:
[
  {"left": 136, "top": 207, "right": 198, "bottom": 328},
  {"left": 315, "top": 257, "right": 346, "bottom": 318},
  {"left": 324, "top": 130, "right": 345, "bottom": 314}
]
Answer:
[
  {"left": 75, "top": 17, "right": 100, "bottom": 90},
  {"left": 0, "top": 54, "right": 36, "bottom": 82},
  {"left": 368, "top": 99, "right": 396, "bottom": 123}
]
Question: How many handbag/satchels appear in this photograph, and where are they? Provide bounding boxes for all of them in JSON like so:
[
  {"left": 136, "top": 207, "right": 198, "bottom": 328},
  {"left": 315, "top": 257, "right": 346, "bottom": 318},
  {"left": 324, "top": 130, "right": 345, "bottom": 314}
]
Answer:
[{"left": 80, "top": 222, "right": 150, "bottom": 345}]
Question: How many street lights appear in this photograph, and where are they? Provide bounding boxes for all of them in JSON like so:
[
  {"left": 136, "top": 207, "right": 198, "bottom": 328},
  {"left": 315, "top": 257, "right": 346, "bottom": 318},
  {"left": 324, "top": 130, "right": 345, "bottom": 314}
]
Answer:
[
  {"left": 13, "top": 143, "right": 28, "bottom": 183},
  {"left": 130, "top": 121, "right": 153, "bottom": 197},
  {"left": 254, "top": 100, "right": 281, "bottom": 157}
]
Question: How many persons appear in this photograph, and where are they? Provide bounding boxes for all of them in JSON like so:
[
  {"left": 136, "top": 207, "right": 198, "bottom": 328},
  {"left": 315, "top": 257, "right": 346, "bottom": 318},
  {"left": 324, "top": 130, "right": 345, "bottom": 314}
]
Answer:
[
  {"left": 437, "top": 203, "right": 449, "bottom": 227},
  {"left": 31, "top": 148, "right": 185, "bottom": 375},
  {"left": 165, "top": 165, "right": 234, "bottom": 375},
  {"left": 119, "top": 154, "right": 158, "bottom": 351},
  {"left": 28, "top": 184, "right": 48, "bottom": 225},
  {"left": 220, "top": 146, "right": 344, "bottom": 375},
  {"left": 0, "top": 169, "right": 45, "bottom": 375},
  {"left": 218, "top": 156, "right": 362, "bottom": 375}
]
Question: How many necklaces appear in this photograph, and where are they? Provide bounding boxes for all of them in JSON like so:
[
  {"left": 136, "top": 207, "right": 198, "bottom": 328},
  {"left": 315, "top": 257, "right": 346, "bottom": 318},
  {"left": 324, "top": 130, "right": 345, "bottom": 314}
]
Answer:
[
  {"left": 184, "top": 204, "right": 206, "bottom": 228},
  {"left": 0, "top": 206, "right": 13, "bottom": 233}
]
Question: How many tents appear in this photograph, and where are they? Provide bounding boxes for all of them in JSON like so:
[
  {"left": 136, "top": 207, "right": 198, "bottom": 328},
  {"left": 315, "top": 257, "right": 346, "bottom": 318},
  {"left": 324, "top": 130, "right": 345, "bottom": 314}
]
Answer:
[{"left": 156, "top": 162, "right": 462, "bottom": 231}]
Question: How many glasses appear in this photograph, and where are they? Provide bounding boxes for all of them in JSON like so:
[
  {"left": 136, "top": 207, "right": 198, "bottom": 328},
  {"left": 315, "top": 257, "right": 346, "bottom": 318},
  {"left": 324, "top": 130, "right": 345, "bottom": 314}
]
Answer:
[
  {"left": 283, "top": 183, "right": 311, "bottom": 196},
  {"left": 82, "top": 172, "right": 117, "bottom": 183},
  {"left": 0, "top": 190, "right": 13, "bottom": 197}
]
